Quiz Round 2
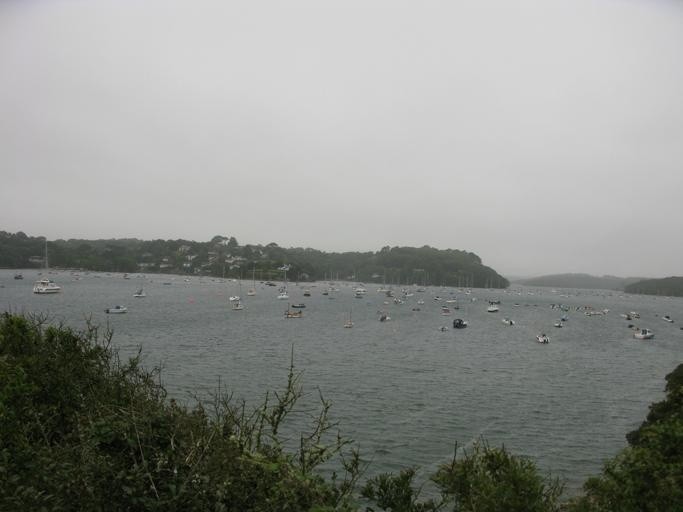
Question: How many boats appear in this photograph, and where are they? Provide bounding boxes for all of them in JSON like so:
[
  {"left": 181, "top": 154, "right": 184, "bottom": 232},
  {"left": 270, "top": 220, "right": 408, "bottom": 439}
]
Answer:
[
  {"left": 134, "top": 288, "right": 146, "bottom": 297},
  {"left": 14, "top": 274, "right": 24, "bottom": 279},
  {"left": 38, "top": 269, "right": 149, "bottom": 282},
  {"left": 618, "top": 310, "right": 655, "bottom": 339},
  {"left": 104, "top": 305, "right": 128, "bottom": 313},
  {"left": 487, "top": 286, "right": 632, "bottom": 328},
  {"left": 661, "top": 315, "right": 674, "bottom": 323},
  {"left": 535, "top": 332, "right": 550, "bottom": 344},
  {"left": 34, "top": 279, "right": 62, "bottom": 294}
]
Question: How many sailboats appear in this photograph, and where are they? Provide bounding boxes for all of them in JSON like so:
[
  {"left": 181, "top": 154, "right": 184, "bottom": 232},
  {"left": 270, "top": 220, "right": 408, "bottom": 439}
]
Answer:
[
  {"left": 217, "top": 268, "right": 317, "bottom": 318},
  {"left": 322, "top": 272, "right": 478, "bottom": 331}
]
